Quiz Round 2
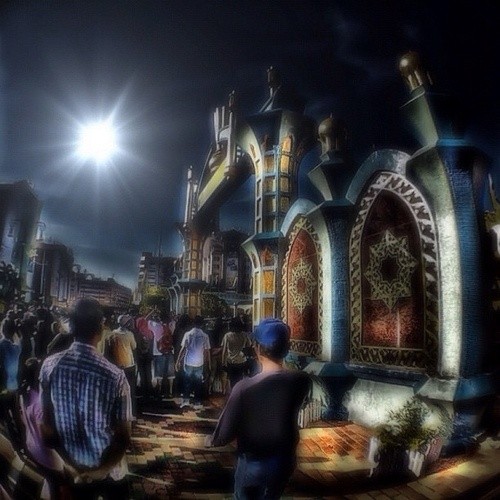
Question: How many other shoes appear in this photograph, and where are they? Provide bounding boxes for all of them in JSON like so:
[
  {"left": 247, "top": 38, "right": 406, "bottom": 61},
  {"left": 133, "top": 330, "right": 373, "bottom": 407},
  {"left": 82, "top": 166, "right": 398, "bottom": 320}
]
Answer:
[
  {"left": 167, "top": 391, "right": 173, "bottom": 397},
  {"left": 179, "top": 401, "right": 190, "bottom": 409}
]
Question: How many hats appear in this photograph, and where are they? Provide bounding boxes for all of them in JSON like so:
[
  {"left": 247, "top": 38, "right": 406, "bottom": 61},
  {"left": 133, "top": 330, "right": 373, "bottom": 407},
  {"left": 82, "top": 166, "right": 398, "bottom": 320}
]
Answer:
[
  {"left": 117, "top": 314, "right": 131, "bottom": 326},
  {"left": 249, "top": 318, "right": 291, "bottom": 347}
]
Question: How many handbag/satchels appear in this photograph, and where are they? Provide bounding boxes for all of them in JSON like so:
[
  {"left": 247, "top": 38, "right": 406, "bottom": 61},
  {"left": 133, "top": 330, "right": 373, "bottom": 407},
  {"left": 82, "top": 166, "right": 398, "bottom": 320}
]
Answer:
[
  {"left": 243, "top": 347, "right": 251, "bottom": 356},
  {"left": 208, "top": 374, "right": 227, "bottom": 396}
]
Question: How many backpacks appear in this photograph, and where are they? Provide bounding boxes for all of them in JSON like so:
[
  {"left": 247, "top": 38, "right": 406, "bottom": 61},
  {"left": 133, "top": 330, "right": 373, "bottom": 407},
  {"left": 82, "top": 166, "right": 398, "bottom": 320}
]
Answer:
[{"left": 157, "top": 327, "right": 174, "bottom": 354}]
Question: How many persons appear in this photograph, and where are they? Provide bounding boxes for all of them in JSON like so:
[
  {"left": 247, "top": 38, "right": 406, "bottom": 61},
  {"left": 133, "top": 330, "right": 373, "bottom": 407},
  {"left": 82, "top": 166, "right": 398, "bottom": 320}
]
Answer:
[
  {"left": 213, "top": 319, "right": 312, "bottom": 500},
  {"left": 17, "top": 359, "right": 67, "bottom": 498},
  {"left": 0, "top": 305, "right": 252, "bottom": 437},
  {"left": 43, "top": 299, "right": 131, "bottom": 499}
]
